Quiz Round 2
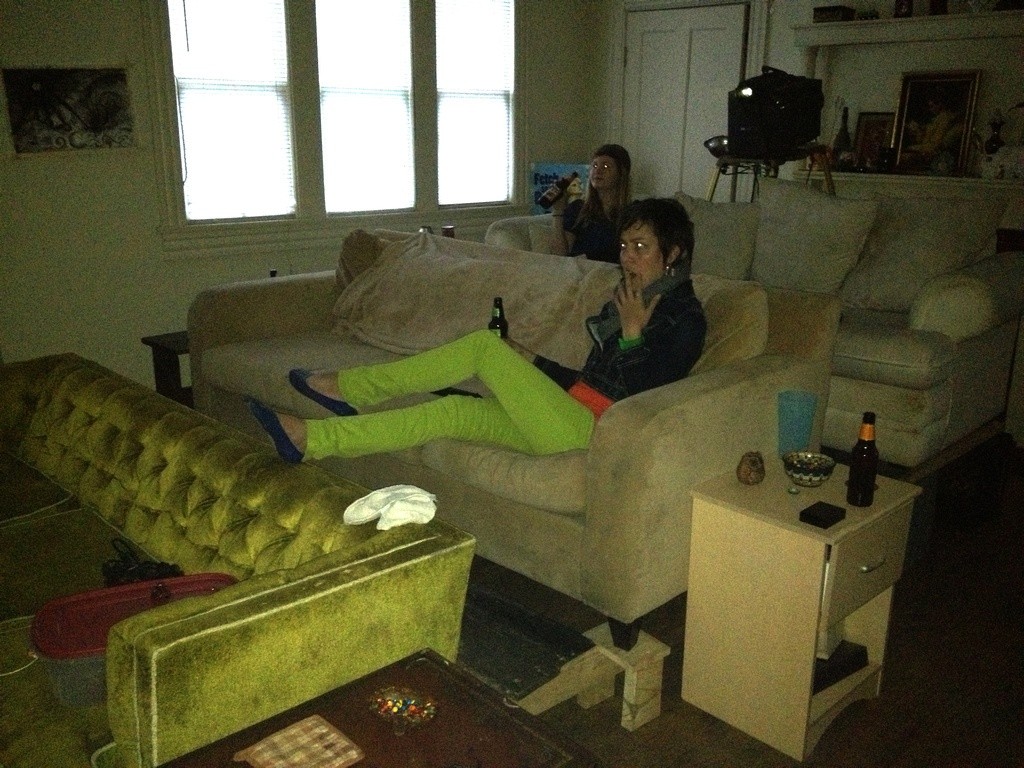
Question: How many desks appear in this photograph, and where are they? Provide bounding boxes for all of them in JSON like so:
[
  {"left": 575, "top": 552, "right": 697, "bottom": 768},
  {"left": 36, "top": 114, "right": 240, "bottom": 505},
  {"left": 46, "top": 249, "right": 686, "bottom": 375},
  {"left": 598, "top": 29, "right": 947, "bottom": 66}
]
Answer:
[{"left": 150, "top": 647, "right": 605, "bottom": 767}]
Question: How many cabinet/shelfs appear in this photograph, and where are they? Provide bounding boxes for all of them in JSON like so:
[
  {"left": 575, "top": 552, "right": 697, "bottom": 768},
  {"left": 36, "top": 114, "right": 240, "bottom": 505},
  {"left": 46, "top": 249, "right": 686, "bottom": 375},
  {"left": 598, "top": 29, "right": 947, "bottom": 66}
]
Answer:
[{"left": 681, "top": 462, "right": 923, "bottom": 763}]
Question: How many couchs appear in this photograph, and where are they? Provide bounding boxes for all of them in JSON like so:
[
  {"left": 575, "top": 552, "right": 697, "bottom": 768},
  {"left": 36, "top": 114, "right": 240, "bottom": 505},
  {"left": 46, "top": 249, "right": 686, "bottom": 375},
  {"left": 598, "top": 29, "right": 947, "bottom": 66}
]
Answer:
[
  {"left": 186, "top": 227, "right": 840, "bottom": 652},
  {"left": 0, "top": 351, "right": 478, "bottom": 768},
  {"left": 485, "top": 206, "right": 1024, "bottom": 468}
]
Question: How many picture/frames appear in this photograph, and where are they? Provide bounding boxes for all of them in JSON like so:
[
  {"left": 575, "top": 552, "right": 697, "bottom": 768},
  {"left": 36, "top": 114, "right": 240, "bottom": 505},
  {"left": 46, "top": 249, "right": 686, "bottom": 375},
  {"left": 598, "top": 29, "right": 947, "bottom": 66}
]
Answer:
[
  {"left": 895, "top": 68, "right": 983, "bottom": 178},
  {"left": 851, "top": 110, "right": 895, "bottom": 171}
]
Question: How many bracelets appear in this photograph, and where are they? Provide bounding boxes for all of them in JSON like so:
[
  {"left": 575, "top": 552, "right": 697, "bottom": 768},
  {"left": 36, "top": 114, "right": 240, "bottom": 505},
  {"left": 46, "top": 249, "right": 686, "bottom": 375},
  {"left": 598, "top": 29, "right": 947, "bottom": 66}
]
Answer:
[{"left": 550, "top": 213, "right": 566, "bottom": 218}]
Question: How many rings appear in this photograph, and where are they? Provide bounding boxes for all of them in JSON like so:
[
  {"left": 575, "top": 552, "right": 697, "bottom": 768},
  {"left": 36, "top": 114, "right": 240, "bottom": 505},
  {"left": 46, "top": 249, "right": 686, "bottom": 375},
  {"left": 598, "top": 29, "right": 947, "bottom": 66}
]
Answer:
[{"left": 634, "top": 290, "right": 644, "bottom": 297}]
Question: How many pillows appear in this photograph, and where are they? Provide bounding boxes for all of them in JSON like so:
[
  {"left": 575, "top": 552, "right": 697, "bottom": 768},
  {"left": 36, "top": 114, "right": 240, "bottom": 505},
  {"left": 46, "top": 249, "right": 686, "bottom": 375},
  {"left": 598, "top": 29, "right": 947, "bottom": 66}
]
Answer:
[
  {"left": 750, "top": 176, "right": 887, "bottom": 295},
  {"left": 837, "top": 195, "right": 1010, "bottom": 312},
  {"left": 671, "top": 187, "right": 761, "bottom": 280}
]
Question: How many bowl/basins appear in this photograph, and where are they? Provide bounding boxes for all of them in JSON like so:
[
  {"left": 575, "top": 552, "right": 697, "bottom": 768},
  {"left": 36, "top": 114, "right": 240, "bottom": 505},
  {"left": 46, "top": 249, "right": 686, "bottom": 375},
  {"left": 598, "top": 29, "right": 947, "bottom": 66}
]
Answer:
[{"left": 782, "top": 452, "right": 836, "bottom": 488}]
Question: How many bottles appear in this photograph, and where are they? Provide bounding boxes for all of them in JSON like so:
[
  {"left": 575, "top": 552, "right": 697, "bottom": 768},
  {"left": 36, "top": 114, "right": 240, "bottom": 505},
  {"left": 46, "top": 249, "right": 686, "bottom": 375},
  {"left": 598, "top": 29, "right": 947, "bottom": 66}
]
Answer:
[
  {"left": 878, "top": 147, "right": 896, "bottom": 173},
  {"left": 846, "top": 412, "right": 879, "bottom": 507},
  {"left": 985, "top": 119, "right": 1005, "bottom": 160},
  {"left": 537, "top": 171, "right": 578, "bottom": 208},
  {"left": 832, "top": 106, "right": 852, "bottom": 171},
  {"left": 488, "top": 297, "right": 508, "bottom": 341}
]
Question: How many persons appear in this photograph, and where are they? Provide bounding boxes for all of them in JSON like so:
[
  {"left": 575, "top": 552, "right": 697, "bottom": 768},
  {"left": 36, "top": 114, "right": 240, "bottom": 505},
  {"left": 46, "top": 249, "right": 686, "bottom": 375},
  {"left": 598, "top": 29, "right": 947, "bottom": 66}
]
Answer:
[
  {"left": 246, "top": 199, "right": 707, "bottom": 462},
  {"left": 548, "top": 144, "right": 632, "bottom": 264}
]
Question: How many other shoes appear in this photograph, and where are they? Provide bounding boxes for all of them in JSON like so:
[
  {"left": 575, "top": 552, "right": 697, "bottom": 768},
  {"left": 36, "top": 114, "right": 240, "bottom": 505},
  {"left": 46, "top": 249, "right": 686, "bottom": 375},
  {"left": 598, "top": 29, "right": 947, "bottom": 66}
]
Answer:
[
  {"left": 253, "top": 401, "right": 304, "bottom": 465},
  {"left": 288, "top": 365, "right": 359, "bottom": 416}
]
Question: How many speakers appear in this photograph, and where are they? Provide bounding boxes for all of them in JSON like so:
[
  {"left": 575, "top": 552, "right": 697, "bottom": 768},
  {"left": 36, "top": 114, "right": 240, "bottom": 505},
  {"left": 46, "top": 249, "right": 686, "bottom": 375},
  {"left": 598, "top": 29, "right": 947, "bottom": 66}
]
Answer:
[{"left": 727, "top": 77, "right": 824, "bottom": 160}]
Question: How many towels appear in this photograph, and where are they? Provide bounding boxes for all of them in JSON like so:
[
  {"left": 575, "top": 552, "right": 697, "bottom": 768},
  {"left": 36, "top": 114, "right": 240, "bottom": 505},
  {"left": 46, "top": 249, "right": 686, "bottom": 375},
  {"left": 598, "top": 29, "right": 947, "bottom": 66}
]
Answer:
[{"left": 340, "top": 483, "right": 438, "bottom": 530}]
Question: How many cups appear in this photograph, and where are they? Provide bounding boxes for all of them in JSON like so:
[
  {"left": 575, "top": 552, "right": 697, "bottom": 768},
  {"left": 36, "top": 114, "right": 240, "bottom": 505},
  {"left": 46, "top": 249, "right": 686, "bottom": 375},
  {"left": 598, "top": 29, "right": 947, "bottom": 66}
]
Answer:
[{"left": 778, "top": 390, "right": 818, "bottom": 459}]
